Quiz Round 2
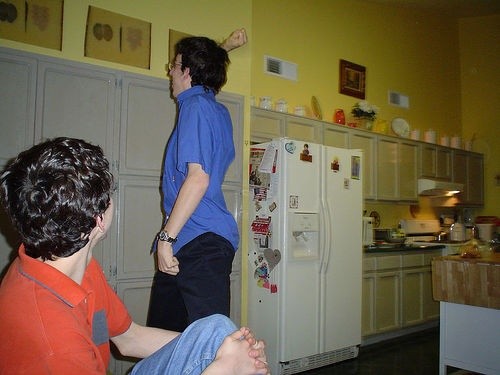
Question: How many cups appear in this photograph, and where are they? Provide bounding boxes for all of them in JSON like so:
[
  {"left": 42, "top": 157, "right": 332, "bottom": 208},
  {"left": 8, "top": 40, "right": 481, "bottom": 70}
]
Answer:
[
  {"left": 294, "top": 106, "right": 306, "bottom": 117},
  {"left": 465, "top": 140, "right": 474, "bottom": 152},
  {"left": 250, "top": 96, "right": 255, "bottom": 106},
  {"left": 424, "top": 130, "right": 437, "bottom": 144},
  {"left": 451, "top": 136, "right": 464, "bottom": 150},
  {"left": 439, "top": 136, "right": 449, "bottom": 147},
  {"left": 275, "top": 99, "right": 288, "bottom": 114},
  {"left": 258, "top": 96, "right": 272, "bottom": 111},
  {"left": 410, "top": 130, "right": 420, "bottom": 141}
]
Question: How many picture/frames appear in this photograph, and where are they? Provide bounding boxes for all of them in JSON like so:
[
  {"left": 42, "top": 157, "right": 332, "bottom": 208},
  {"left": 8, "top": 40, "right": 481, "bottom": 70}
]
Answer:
[{"left": 338, "top": 58, "right": 367, "bottom": 101}]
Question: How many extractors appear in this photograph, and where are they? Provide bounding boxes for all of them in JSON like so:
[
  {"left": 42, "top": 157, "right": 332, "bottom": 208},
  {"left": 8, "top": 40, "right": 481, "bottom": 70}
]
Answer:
[{"left": 417, "top": 177, "right": 464, "bottom": 197}]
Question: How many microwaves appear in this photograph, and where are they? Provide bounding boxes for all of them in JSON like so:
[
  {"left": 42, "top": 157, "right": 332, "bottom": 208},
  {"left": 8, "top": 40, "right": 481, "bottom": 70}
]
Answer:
[{"left": 362, "top": 217, "right": 373, "bottom": 247}]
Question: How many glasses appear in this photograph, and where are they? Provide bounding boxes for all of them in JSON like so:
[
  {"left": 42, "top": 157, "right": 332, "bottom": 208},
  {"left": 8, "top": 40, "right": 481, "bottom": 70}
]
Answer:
[{"left": 168, "top": 62, "right": 182, "bottom": 69}]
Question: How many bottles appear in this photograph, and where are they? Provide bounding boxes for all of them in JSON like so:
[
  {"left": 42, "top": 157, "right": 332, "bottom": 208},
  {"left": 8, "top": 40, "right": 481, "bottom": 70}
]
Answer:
[
  {"left": 471, "top": 225, "right": 479, "bottom": 240},
  {"left": 334, "top": 109, "right": 346, "bottom": 125}
]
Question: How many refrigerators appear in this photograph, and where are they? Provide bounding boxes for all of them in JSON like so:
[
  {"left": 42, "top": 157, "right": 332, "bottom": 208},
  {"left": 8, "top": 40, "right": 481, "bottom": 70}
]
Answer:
[{"left": 247, "top": 137, "right": 364, "bottom": 375}]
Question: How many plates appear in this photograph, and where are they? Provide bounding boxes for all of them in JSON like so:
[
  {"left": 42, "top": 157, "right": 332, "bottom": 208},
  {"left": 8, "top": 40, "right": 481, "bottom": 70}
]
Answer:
[
  {"left": 310, "top": 96, "right": 322, "bottom": 120},
  {"left": 391, "top": 118, "right": 410, "bottom": 136}
]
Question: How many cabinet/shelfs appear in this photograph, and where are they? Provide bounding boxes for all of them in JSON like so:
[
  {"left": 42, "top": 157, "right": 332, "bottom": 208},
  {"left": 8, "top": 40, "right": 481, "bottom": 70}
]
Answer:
[
  {"left": 250, "top": 107, "right": 485, "bottom": 209},
  {"left": 0, "top": 45, "right": 245, "bottom": 375},
  {"left": 359, "top": 247, "right": 441, "bottom": 348}
]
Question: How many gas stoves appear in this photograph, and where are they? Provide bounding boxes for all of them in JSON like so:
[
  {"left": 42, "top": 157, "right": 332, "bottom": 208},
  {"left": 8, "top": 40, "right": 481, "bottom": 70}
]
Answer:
[{"left": 399, "top": 219, "right": 466, "bottom": 247}]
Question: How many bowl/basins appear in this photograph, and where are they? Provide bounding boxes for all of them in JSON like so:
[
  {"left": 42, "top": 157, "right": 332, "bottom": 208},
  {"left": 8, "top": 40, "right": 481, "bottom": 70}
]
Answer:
[{"left": 386, "top": 238, "right": 405, "bottom": 243}]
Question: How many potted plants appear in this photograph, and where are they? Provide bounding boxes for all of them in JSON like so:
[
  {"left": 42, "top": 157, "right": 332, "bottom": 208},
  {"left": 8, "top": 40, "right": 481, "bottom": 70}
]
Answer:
[{"left": 351, "top": 101, "right": 377, "bottom": 130}]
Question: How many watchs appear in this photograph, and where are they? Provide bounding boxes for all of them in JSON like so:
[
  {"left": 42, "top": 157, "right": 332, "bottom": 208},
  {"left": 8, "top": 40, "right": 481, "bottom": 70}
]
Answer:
[{"left": 158, "top": 230, "right": 177, "bottom": 243}]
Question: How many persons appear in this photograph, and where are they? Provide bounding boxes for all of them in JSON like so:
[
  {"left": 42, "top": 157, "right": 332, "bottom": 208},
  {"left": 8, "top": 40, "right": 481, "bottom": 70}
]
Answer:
[
  {"left": 0, "top": 138, "right": 269, "bottom": 375},
  {"left": 145, "top": 28, "right": 248, "bottom": 334}
]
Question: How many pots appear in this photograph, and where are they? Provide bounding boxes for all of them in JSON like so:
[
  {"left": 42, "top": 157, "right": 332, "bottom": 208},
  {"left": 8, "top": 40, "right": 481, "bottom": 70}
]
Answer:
[
  {"left": 434, "top": 230, "right": 447, "bottom": 241},
  {"left": 475, "top": 215, "right": 500, "bottom": 242},
  {"left": 449, "top": 223, "right": 466, "bottom": 242}
]
